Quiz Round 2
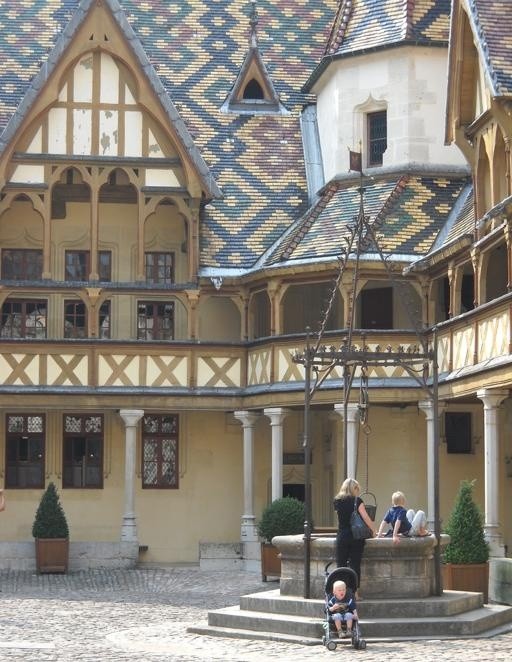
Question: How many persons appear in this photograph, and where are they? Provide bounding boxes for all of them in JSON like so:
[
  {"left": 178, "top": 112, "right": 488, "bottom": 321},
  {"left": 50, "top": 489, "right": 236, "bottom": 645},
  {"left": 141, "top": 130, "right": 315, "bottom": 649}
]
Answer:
[
  {"left": 332, "top": 477, "right": 376, "bottom": 601},
  {"left": 327, "top": 580, "right": 358, "bottom": 638},
  {"left": 378, "top": 491, "right": 431, "bottom": 545}
]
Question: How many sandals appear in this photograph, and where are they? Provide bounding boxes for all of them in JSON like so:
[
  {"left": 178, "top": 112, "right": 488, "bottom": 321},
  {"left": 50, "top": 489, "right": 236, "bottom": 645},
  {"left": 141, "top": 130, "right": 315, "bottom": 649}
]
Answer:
[{"left": 418, "top": 530, "right": 432, "bottom": 536}]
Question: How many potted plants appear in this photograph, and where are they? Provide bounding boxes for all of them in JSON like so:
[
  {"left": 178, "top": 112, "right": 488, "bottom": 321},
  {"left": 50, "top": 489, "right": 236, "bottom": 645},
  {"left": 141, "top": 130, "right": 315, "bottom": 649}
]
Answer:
[
  {"left": 257, "top": 494, "right": 313, "bottom": 581},
  {"left": 32, "top": 482, "right": 69, "bottom": 573},
  {"left": 439, "top": 478, "right": 487, "bottom": 604}
]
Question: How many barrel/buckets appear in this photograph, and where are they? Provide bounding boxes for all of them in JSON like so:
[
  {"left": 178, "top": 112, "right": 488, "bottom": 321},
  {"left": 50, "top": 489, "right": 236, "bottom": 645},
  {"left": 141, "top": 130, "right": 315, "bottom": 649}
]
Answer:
[{"left": 358, "top": 492, "right": 376, "bottom": 523}]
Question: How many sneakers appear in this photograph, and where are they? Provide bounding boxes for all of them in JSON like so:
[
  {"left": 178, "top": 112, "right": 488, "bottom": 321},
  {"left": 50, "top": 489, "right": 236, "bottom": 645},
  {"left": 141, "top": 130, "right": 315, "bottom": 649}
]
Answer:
[{"left": 338, "top": 629, "right": 352, "bottom": 638}]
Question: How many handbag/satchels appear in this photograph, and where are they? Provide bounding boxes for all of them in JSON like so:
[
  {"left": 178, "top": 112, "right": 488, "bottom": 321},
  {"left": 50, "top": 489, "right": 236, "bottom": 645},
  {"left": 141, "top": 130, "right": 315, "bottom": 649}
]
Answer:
[{"left": 350, "top": 512, "right": 373, "bottom": 539}]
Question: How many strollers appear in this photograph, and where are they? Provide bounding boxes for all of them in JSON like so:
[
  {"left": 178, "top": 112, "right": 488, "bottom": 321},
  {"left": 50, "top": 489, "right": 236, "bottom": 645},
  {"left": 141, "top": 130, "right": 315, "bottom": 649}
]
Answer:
[{"left": 318, "top": 558, "right": 367, "bottom": 652}]
